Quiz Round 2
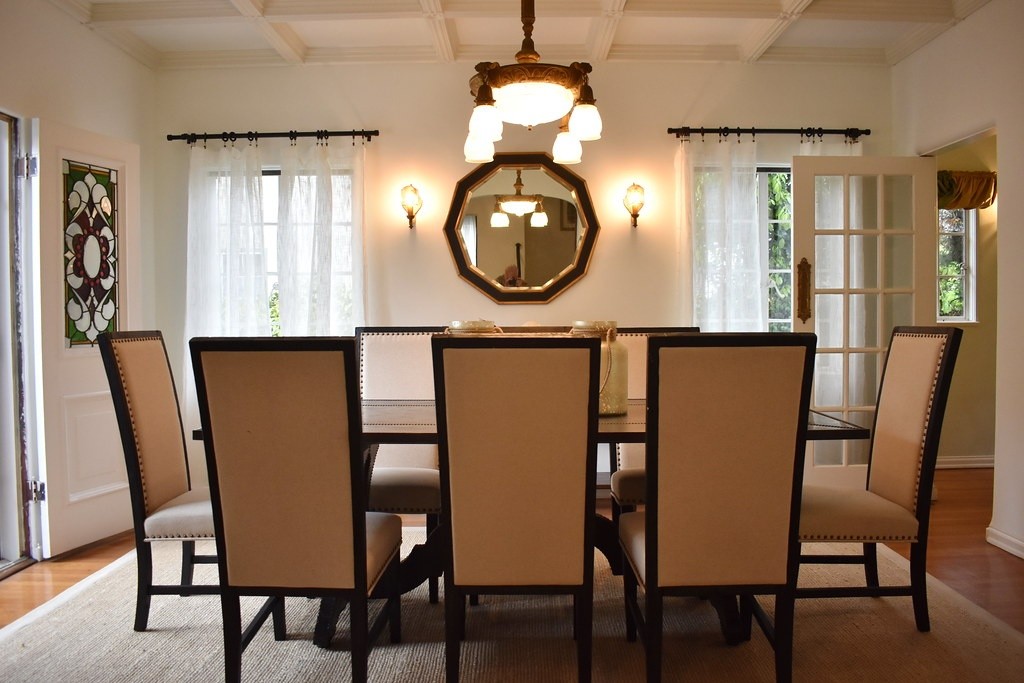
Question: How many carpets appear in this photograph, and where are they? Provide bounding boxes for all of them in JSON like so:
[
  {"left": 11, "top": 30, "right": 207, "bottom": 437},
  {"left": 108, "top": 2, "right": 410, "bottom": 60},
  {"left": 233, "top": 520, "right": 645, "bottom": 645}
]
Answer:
[{"left": 0, "top": 527, "right": 1024, "bottom": 683}]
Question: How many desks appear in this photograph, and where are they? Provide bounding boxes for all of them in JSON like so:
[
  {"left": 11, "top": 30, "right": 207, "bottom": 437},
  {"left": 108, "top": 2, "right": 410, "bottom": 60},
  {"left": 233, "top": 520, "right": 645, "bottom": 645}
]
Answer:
[{"left": 191, "top": 399, "right": 870, "bottom": 645}]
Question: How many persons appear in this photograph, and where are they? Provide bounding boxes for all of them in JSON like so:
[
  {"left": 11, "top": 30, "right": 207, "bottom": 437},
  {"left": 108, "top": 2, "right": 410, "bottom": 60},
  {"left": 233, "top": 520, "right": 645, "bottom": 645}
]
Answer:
[{"left": 496, "top": 265, "right": 527, "bottom": 287}]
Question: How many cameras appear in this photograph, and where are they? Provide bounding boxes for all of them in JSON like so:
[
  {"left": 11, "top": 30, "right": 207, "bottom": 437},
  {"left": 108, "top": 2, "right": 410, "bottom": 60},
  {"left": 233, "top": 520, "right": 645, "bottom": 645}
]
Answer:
[{"left": 509, "top": 276, "right": 517, "bottom": 285}]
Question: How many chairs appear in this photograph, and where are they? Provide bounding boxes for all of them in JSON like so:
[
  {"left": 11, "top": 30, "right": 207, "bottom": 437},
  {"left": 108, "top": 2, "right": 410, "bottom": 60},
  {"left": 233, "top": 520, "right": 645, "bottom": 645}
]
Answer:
[
  {"left": 795, "top": 325, "right": 964, "bottom": 633},
  {"left": 609, "top": 328, "right": 701, "bottom": 524},
  {"left": 354, "top": 326, "right": 452, "bottom": 605},
  {"left": 96, "top": 330, "right": 216, "bottom": 632},
  {"left": 430, "top": 332, "right": 601, "bottom": 683},
  {"left": 188, "top": 335, "right": 404, "bottom": 683},
  {"left": 619, "top": 332, "right": 818, "bottom": 683}
]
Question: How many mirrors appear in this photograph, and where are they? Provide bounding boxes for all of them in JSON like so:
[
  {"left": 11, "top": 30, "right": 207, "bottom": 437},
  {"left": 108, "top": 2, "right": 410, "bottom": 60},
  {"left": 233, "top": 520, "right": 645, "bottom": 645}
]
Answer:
[{"left": 442, "top": 151, "right": 602, "bottom": 306}]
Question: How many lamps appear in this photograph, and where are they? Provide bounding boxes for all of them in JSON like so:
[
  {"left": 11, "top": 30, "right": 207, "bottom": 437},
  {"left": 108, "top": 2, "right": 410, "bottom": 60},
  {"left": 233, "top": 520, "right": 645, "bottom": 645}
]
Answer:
[
  {"left": 400, "top": 184, "right": 424, "bottom": 229},
  {"left": 464, "top": 0, "right": 603, "bottom": 166},
  {"left": 489, "top": 170, "right": 549, "bottom": 228},
  {"left": 622, "top": 183, "right": 645, "bottom": 228}
]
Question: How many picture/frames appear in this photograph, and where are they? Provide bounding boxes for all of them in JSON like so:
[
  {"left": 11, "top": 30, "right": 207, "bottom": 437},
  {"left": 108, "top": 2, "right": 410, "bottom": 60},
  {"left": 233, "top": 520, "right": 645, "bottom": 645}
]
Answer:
[{"left": 559, "top": 199, "right": 576, "bottom": 231}]
